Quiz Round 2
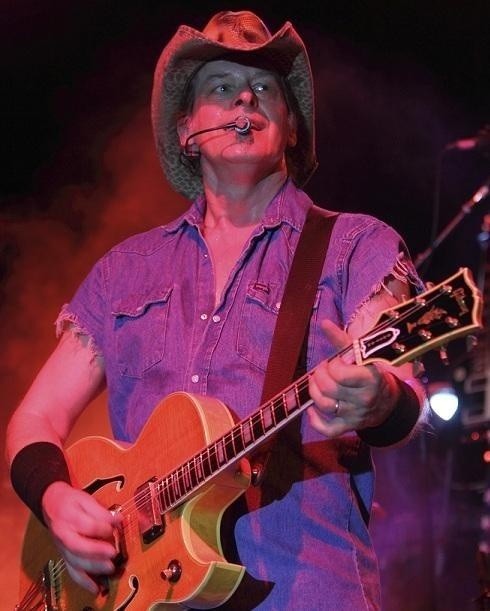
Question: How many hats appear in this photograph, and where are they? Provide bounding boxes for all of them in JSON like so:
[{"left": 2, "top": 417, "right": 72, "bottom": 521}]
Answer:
[{"left": 150, "top": 9, "right": 319, "bottom": 201}]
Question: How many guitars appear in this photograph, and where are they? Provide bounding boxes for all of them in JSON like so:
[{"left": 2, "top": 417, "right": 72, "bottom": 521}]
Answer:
[{"left": 16, "top": 266, "right": 484, "bottom": 611}]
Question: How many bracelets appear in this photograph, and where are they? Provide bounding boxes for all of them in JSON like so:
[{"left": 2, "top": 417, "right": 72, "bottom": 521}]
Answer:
[{"left": 11, "top": 443, "right": 70, "bottom": 530}]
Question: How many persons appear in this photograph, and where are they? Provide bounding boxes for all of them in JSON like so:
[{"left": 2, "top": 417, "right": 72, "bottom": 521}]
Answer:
[{"left": 3, "top": 10, "right": 430, "bottom": 611}]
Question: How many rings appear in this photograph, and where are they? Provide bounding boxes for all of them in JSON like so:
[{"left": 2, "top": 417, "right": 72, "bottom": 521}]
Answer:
[{"left": 333, "top": 399, "right": 340, "bottom": 416}]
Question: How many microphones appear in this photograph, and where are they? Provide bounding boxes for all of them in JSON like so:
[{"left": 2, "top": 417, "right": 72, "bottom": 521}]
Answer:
[{"left": 185, "top": 116, "right": 251, "bottom": 151}]
